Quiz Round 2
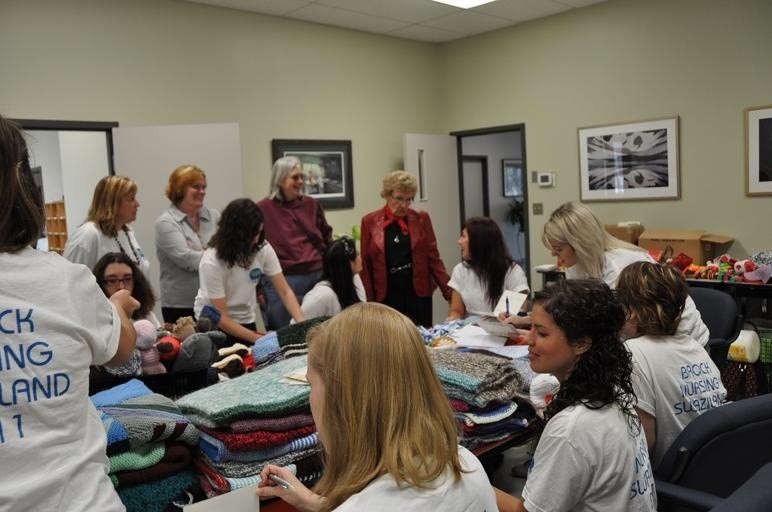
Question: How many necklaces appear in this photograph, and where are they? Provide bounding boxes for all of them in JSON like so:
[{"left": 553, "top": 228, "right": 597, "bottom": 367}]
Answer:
[
  {"left": 389, "top": 223, "right": 405, "bottom": 243},
  {"left": 107, "top": 224, "right": 140, "bottom": 265}
]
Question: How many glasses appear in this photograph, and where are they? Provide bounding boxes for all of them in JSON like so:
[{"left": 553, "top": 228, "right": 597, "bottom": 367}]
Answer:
[
  {"left": 391, "top": 194, "right": 412, "bottom": 204},
  {"left": 103, "top": 277, "right": 133, "bottom": 286},
  {"left": 288, "top": 174, "right": 304, "bottom": 180}
]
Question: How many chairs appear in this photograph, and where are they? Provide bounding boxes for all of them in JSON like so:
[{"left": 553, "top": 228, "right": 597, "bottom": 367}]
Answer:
[
  {"left": 682, "top": 284, "right": 742, "bottom": 380},
  {"left": 651, "top": 463, "right": 772, "bottom": 512},
  {"left": 649, "top": 391, "right": 772, "bottom": 499}
]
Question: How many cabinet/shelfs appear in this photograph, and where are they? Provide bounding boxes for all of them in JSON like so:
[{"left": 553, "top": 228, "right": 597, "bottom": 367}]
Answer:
[
  {"left": 42, "top": 200, "right": 66, "bottom": 258},
  {"left": 684, "top": 278, "right": 771, "bottom": 393}
]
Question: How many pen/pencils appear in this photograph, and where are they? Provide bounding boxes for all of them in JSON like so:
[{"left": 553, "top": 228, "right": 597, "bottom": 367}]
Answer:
[
  {"left": 504, "top": 295, "right": 511, "bottom": 320},
  {"left": 257, "top": 466, "right": 296, "bottom": 495}
]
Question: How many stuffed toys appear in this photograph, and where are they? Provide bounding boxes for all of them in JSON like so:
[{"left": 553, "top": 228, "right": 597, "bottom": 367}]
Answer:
[{"left": 105, "top": 305, "right": 225, "bottom": 394}]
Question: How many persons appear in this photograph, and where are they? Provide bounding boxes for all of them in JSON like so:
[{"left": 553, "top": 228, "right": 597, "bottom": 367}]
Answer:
[
  {"left": 445, "top": 216, "right": 531, "bottom": 322},
  {"left": 510, "top": 261, "right": 727, "bottom": 478},
  {"left": 64, "top": 175, "right": 161, "bottom": 331},
  {"left": 195, "top": 199, "right": 305, "bottom": 348},
  {"left": 498, "top": 200, "right": 711, "bottom": 357},
  {"left": 155, "top": 165, "right": 222, "bottom": 323},
  {"left": 257, "top": 156, "right": 332, "bottom": 329},
  {"left": 92, "top": 253, "right": 162, "bottom": 332},
  {"left": 254, "top": 301, "right": 499, "bottom": 512},
  {"left": 1, "top": 118, "right": 140, "bottom": 512},
  {"left": 290, "top": 236, "right": 365, "bottom": 326},
  {"left": 493, "top": 277, "right": 658, "bottom": 512},
  {"left": 361, "top": 170, "right": 453, "bottom": 329}
]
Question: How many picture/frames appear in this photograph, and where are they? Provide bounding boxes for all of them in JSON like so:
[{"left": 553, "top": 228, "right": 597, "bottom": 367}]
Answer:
[
  {"left": 743, "top": 104, "right": 772, "bottom": 197},
  {"left": 501, "top": 159, "right": 523, "bottom": 198},
  {"left": 273, "top": 139, "right": 355, "bottom": 210},
  {"left": 576, "top": 115, "right": 680, "bottom": 203}
]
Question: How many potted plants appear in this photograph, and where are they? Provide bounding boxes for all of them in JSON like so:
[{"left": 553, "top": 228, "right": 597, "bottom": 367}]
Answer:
[{"left": 505, "top": 199, "right": 526, "bottom": 275}]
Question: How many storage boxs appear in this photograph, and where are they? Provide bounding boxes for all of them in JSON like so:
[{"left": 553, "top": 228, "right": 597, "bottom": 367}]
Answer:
[
  {"left": 604, "top": 224, "right": 638, "bottom": 246},
  {"left": 639, "top": 230, "right": 738, "bottom": 267}
]
